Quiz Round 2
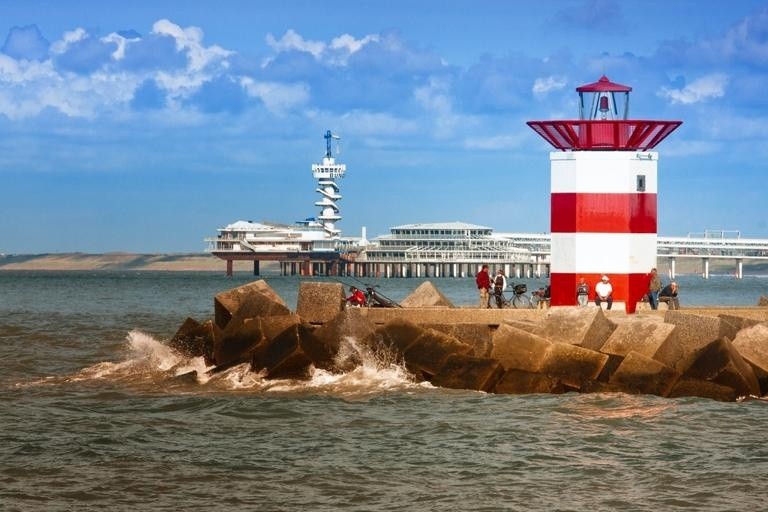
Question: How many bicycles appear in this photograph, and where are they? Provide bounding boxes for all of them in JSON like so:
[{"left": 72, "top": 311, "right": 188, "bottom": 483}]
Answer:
[
  {"left": 487, "top": 280, "right": 532, "bottom": 309},
  {"left": 363, "top": 282, "right": 381, "bottom": 306}
]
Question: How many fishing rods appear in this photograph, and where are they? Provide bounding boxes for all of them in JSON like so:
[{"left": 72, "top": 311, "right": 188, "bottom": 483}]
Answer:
[{"left": 319, "top": 267, "right": 403, "bottom": 308}]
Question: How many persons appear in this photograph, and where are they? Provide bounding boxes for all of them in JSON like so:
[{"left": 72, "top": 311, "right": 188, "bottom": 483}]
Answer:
[
  {"left": 595, "top": 275, "right": 613, "bottom": 310},
  {"left": 659, "top": 282, "right": 680, "bottom": 310},
  {"left": 346, "top": 286, "right": 367, "bottom": 308},
  {"left": 492, "top": 270, "right": 507, "bottom": 309},
  {"left": 576, "top": 277, "right": 590, "bottom": 307},
  {"left": 476, "top": 265, "right": 491, "bottom": 308},
  {"left": 649, "top": 268, "right": 661, "bottom": 310}
]
